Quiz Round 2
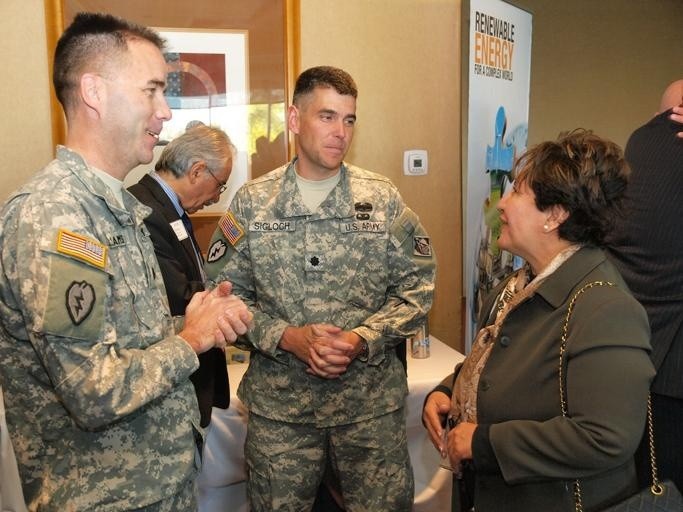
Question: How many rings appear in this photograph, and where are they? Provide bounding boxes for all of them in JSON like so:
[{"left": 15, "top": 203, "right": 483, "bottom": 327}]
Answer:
[
  {"left": 247, "top": 310, "right": 253, "bottom": 324},
  {"left": 343, "top": 349, "right": 347, "bottom": 357}
]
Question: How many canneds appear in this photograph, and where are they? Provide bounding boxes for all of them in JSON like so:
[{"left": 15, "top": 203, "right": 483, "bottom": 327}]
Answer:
[{"left": 409, "top": 318, "right": 431, "bottom": 361}]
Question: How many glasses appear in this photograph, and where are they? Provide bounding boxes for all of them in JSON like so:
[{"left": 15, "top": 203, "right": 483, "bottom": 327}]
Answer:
[{"left": 206, "top": 166, "right": 227, "bottom": 195}]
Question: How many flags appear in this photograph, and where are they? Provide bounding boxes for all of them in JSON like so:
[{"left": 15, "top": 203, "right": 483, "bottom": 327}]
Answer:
[{"left": 161, "top": 50, "right": 231, "bottom": 112}]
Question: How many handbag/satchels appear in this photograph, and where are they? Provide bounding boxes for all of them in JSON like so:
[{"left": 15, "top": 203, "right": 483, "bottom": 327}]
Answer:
[{"left": 600, "top": 479, "right": 683, "bottom": 512}]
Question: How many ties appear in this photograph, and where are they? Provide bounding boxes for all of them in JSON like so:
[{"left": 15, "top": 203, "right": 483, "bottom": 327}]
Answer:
[{"left": 181, "top": 211, "right": 205, "bottom": 266}]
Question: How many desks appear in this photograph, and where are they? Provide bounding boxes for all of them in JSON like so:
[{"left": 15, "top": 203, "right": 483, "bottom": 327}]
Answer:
[{"left": 192, "top": 333, "right": 465, "bottom": 512}]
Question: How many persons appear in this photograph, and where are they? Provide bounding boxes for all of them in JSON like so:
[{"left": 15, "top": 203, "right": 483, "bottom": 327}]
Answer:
[
  {"left": 591, "top": 101, "right": 682, "bottom": 501},
  {"left": 0, "top": 10, "right": 256, "bottom": 512},
  {"left": 201, "top": 65, "right": 439, "bottom": 510},
  {"left": 653, "top": 78, "right": 682, "bottom": 119},
  {"left": 419, "top": 127, "right": 657, "bottom": 511},
  {"left": 123, "top": 121, "right": 235, "bottom": 443}
]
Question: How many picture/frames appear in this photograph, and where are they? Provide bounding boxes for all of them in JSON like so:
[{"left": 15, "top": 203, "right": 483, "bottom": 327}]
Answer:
[{"left": 42, "top": 0, "right": 300, "bottom": 256}]
